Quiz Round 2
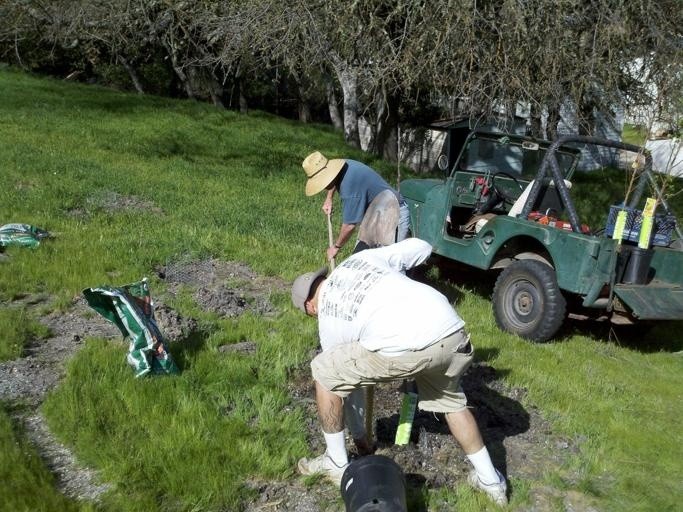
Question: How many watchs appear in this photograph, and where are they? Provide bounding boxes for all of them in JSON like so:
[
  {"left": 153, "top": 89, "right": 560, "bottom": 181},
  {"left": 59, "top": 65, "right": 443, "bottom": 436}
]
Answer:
[{"left": 334, "top": 244, "right": 341, "bottom": 249}]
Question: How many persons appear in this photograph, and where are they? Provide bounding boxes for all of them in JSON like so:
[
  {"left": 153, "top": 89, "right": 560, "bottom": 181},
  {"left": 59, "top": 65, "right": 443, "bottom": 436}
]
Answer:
[
  {"left": 302, "top": 151, "right": 410, "bottom": 261},
  {"left": 291, "top": 237, "right": 509, "bottom": 508}
]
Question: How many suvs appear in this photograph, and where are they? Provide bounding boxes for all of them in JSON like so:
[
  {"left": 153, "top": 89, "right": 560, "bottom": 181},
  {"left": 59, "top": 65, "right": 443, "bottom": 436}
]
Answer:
[{"left": 391, "top": 127, "right": 683, "bottom": 349}]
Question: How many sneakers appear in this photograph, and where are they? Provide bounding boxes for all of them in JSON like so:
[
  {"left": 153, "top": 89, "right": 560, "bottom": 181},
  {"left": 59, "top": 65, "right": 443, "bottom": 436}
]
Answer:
[
  {"left": 467, "top": 466, "right": 511, "bottom": 508},
  {"left": 298, "top": 452, "right": 350, "bottom": 490}
]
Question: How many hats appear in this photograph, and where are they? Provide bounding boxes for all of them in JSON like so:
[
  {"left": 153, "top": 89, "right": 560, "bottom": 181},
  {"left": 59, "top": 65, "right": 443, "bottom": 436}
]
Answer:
[
  {"left": 290, "top": 264, "right": 328, "bottom": 317},
  {"left": 301, "top": 150, "right": 346, "bottom": 197}
]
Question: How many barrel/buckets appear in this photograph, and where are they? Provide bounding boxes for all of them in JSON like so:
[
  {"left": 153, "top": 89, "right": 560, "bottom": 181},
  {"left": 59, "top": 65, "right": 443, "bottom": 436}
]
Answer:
[
  {"left": 623, "top": 246, "right": 655, "bottom": 285},
  {"left": 615, "top": 252, "right": 628, "bottom": 283},
  {"left": 340, "top": 454, "right": 408, "bottom": 512}
]
